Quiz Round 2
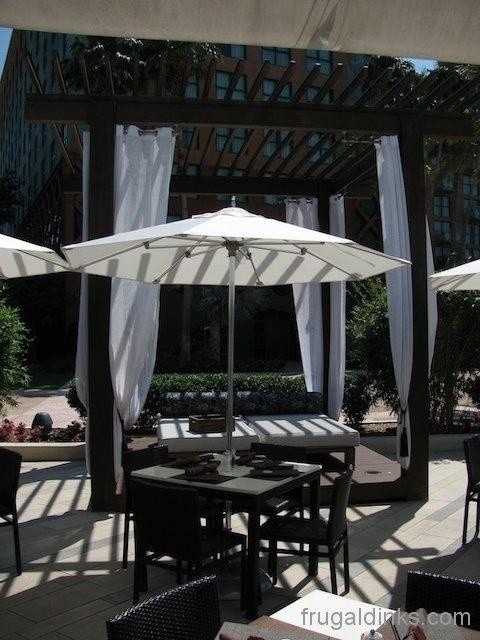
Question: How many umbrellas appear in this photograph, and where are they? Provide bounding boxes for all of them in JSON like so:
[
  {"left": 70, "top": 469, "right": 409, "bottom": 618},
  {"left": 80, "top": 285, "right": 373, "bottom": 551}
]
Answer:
[
  {"left": 0, "top": 232, "right": 69, "bottom": 280},
  {"left": 62, "top": 194, "right": 413, "bottom": 530},
  {"left": 428, "top": 257, "right": 479, "bottom": 293}
]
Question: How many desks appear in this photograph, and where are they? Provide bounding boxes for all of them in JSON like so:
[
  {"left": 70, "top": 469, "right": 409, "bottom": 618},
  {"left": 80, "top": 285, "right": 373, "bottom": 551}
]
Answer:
[
  {"left": 247, "top": 412, "right": 361, "bottom": 472},
  {"left": 156, "top": 415, "right": 258, "bottom": 463}
]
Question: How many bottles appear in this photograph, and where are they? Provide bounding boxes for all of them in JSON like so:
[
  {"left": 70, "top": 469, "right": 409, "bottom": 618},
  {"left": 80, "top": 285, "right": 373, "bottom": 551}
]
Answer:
[
  {"left": 231, "top": 451, "right": 235, "bottom": 469},
  {"left": 223, "top": 452, "right": 231, "bottom": 471}
]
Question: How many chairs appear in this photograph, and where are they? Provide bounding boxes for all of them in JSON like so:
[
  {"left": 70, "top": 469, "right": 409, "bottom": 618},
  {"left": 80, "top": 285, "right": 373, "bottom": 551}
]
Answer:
[
  {"left": 406, "top": 538, "right": 480, "bottom": 631},
  {"left": 460, "top": 434, "right": 479, "bottom": 544},
  {"left": 0, "top": 445, "right": 23, "bottom": 577},
  {"left": 121, "top": 445, "right": 207, "bottom": 583},
  {"left": 105, "top": 574, "right": 223, "bottom": 638},
  {"left": 127, "top": 475, "right": 247, "bottom": 611},
  {"left": 258, "top": 471, "right": 354, "bottom": 595}
]
